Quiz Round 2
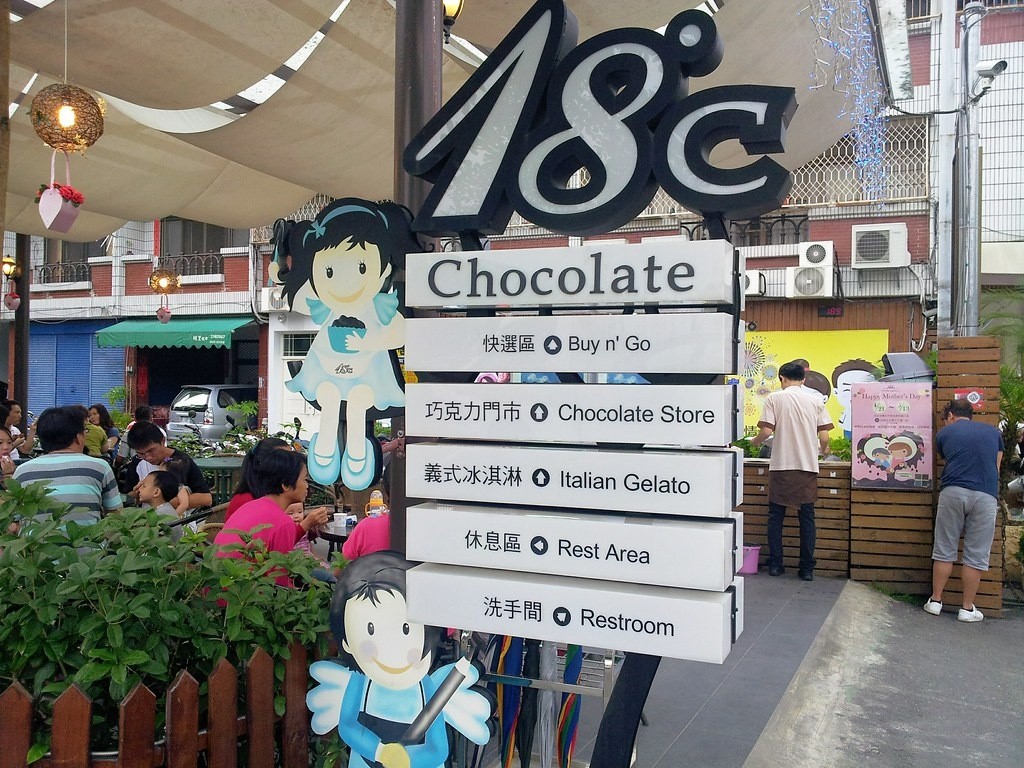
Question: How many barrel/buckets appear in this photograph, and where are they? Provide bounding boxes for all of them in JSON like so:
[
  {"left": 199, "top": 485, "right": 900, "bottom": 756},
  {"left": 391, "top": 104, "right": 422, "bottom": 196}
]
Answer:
[{"left": 736, "top": 547, "right": 760, "bottom": 574}]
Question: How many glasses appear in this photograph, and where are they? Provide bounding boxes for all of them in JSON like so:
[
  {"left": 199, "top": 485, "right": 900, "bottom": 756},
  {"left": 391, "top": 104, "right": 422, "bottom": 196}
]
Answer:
[
  {"left": 136, "top": 440, "right": 162, "bottom": 460},
  {"left": 83, "top": 427, "right": 91, "bottom": 433}
]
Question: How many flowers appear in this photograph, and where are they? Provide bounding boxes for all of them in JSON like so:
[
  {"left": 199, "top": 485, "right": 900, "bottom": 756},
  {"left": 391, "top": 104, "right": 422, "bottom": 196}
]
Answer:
[{"left": 34, "top": 184, "right": 85, "bottom": 207}]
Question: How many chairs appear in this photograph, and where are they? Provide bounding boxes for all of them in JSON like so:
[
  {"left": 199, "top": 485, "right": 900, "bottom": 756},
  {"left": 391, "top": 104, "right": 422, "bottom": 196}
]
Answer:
[{"left": 195, "top": 522, "right": 225, "bottom": 547}]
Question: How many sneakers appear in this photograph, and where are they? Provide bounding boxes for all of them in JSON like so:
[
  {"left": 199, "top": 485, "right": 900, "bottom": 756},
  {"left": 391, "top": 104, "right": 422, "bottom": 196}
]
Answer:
[
  {"left": 958, "top": 602, "right": 984, "bottom": 622},
  {"left": 923, "top": 597, "right": 943, "bottom": 615}
]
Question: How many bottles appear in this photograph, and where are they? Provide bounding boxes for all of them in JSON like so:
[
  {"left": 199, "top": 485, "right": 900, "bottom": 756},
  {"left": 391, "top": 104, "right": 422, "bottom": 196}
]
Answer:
[{"left": 365, "top": 490, "right": 388, "bottom": 517}]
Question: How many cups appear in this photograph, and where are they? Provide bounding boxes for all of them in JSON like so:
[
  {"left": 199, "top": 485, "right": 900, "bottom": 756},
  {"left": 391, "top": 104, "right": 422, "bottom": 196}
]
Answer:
[{"left": 333, "top": 513, "right": 346, "bottom": 527}]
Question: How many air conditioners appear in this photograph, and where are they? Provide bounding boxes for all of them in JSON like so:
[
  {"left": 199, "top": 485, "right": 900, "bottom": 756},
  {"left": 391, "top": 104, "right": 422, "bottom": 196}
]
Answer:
[
  {"left": 786, "top": 266, "right": 833, "bottom": 299},
  {"left": 745, "top": 270, "right": 759, "bottom": 296},
  {"left": 851, "top": 223, "right": 911, "bottom": 269},
  {"left": 798, "top": 240, "right": 833, "bottom": 267}
]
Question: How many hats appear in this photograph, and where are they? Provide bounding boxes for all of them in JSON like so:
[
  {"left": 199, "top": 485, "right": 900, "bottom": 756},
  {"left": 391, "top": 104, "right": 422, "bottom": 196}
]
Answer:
[{"left": 998, "top": 418, "right": 1024, "bottom": 433}]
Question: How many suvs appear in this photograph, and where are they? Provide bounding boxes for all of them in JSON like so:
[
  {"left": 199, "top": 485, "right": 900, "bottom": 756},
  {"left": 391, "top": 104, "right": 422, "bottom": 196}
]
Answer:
[{"left": 163, "top": 383, "right": 258, "bottom": 447}]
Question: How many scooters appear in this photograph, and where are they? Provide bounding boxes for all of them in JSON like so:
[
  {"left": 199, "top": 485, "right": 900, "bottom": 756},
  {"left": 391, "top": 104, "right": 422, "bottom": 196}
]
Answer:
[{"left": 176, "top": 410, "right": 311, "bottom": 457}]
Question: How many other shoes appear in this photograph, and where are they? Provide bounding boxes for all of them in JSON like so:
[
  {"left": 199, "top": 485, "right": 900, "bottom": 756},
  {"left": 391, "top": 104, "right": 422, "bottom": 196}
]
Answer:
[
  {"left": 769, "top": 565, "right": 785, "bottom": 576},
  {"left": 797, "top": 569, "right": 814, "bottom": 580}
]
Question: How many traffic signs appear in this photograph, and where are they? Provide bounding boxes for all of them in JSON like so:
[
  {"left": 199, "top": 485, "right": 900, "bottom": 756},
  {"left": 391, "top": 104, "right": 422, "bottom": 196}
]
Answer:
[
  {"left": 403, "top": 312, "right": 746, "bottom": 374},
  {"left": 404, "top": 562, "right": 745, "bottom": 666},
  {"left": 403, "top": 499, "right": 745, "bottom": 592},
  {"left": 403, "top": 381, "right": 745, "bottom": 447},
  {"left": 404, "top": 441, "right": 743, "bottom": 519}
]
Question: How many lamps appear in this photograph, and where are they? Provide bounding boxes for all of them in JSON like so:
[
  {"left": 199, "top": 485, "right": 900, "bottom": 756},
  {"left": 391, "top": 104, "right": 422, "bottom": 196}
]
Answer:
[
  {"left": 32, "top": 0, "right": 104, "bottom": 152},
  {"left": 150, "top": 268, "right": 177, "bottom": 294}
]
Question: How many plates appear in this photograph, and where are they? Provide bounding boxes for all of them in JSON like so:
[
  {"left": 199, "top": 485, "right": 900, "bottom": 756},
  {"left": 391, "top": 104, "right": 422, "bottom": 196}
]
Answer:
[{"left": 967, "top": 392, "right": 980, "bottom": 403}]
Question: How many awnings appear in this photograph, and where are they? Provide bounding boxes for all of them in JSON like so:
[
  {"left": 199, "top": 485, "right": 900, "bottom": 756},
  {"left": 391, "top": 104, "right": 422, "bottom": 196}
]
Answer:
[{"left": 96, "top": 317, "right": 254, "bottom": 349}]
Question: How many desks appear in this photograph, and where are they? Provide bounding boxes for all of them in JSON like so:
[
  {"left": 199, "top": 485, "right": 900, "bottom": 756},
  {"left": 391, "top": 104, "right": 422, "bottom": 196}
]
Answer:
[
  {"left": 315, "top": 518, "right": 361, "bottom": 561},
  {"left": 195, "top": 457, "right": 244, "bottom": 506}
]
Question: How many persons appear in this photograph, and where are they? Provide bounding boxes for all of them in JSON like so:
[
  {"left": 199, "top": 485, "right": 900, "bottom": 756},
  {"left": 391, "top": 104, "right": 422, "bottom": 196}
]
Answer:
[
  {"left": 285, "top": 500, "right": 330, "bottom": 569},
  {"left": 341, "top": 462, "right": 391, "bottom": 560},
  {"left": 0, "top": 426, "right": 17, "bottom": 478},
  {"left": 751, "top": 362, "right": 834, "bottom": 580},
  {"left": 924, "top": 400, "right": 1005, "bottom": 622},
  {"left": 225, "top": 438, "right": 338, "bottom": 581},
  {"left": 139, "top": 470, "right": 183, "bottom": 544},
  {"left": 205, "top": 448, "right": 308, "bottom": 607},
  {"left": 3, "top": 400, "right": 38, "bottom": 458},
  {"left": 88, "top": 403, "right": 121, "bottom": 459},
  {"left": 123, "top": 421, "right": 212, "bottom": 535},
  {"left": 9, "top": 407, "right": 123, "bottom": 566},
  {"left": 73, "top": 404, "right": 111, "bottom": 457},
  {"left": 115, "top": 406, "right": 168, "bottom": 493}
]
